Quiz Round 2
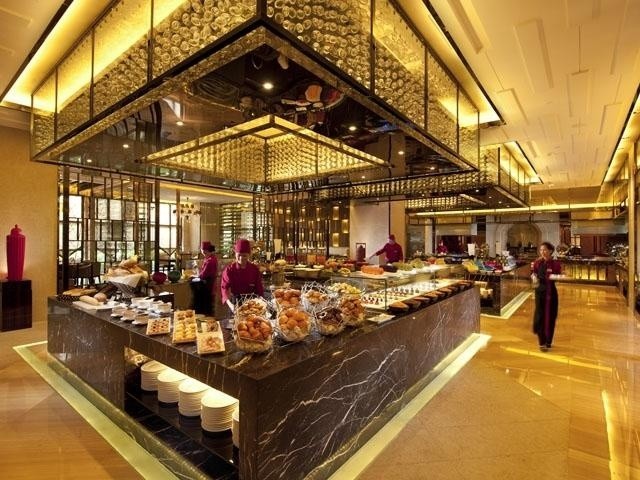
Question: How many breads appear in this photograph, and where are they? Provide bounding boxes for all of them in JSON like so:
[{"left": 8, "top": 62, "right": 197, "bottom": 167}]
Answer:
[{"left": 149, "top": 282, "right": 363, "bottom": 352}]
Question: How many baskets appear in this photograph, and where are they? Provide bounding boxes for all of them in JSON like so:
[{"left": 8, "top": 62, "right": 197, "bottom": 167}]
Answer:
[
  {"left": 110, "top": 277, "right": 145, "bottom": 299},
  {"left": 232, "top": 270, "right": 364, "bottom": 353}
]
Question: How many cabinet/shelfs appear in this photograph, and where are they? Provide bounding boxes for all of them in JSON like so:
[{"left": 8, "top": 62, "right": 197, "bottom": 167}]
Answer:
[{"left": 0, "top": 277, "right": 35, "bottom": 332}]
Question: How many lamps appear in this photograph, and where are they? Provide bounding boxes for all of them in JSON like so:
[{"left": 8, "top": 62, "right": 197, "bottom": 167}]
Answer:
[{"left": 172, "top": 190, "right": 202, "bottom": 224}]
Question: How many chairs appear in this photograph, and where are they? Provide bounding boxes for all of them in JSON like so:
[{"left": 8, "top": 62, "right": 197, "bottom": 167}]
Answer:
[{"left": 59, "top": 259, "right": 101, "bottom": 287}]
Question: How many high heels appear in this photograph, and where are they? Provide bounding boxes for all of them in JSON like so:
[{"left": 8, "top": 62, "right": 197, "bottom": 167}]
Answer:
[{"left": 540, "top": 344, "right": 551, "bottom": 352}]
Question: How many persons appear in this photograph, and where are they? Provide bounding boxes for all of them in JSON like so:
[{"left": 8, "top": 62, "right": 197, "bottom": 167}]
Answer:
[
  {"left": 369, "top": 235, "right": 403, "bottom": 264},
  {"left": 220, "top": 239, "right": 264, "bottom": 319},
  {"left": 189, "top": 241, "right": 218, "bottom": 316},
  {"left": 437, "top": 240, "right": 448, "bottom": 253},
  {"left": 530, "top": 243, "right": 566, "bottom": 348}
]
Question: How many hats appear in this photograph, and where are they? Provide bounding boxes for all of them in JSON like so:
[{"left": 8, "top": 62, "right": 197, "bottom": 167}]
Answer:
[
  {"left": 235, "top": 240, "right": 250, "bottom": 253},
  {"left": 201, "top": 241, "right": 211, "bottom": 250},
  {"left": 439, "top": 240, "right": 443, "bottom": 243},
  {"left": 389, "top": 235, "right": 395, "bottom": 240}
]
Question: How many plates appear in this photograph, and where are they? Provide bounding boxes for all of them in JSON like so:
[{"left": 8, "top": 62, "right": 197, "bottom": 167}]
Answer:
[{"left": 140, "top": 360, "right": 240, "bottom": 449}]
